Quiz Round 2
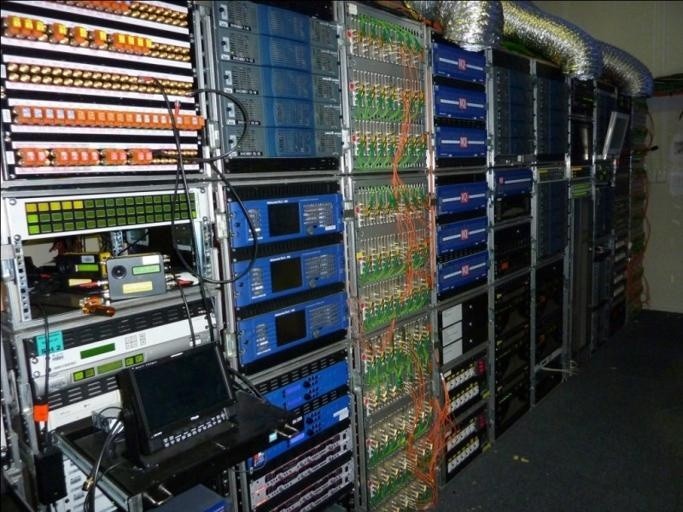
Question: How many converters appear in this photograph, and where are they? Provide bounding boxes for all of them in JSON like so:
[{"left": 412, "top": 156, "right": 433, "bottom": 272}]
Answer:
[{"left": 33, "top": 446, "right": 67, "bottom": 506}]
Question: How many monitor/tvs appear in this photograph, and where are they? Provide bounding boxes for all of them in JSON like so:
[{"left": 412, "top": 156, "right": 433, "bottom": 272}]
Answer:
[
  {"left": 117, "top": 340, "right": 239, "bottom": 456},
  {"left": 602, "top": 112, "right": 630, "bottom": 160}
]
